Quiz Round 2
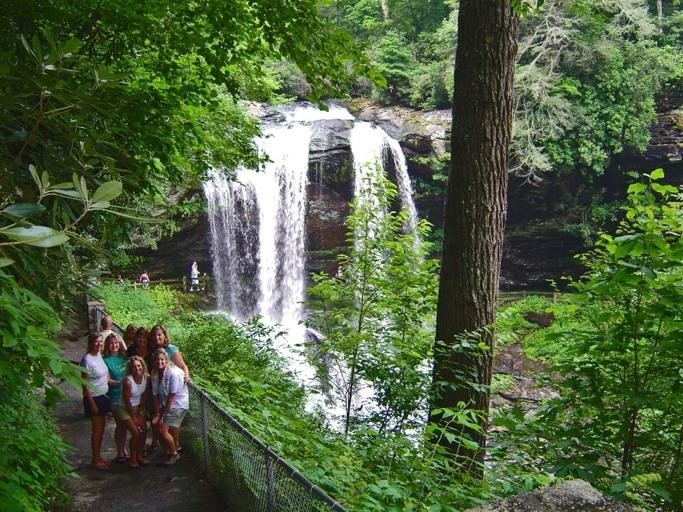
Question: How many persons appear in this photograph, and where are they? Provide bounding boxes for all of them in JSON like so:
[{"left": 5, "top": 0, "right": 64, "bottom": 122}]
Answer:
[
  {"left": 140, "top": 270, "right": 149, "bottom": 288},
  {"left": 337, "top": 264, "right": 344, "bottom": 276},
  {"left": 190, "top": 261, "right": 201, "bottom": 291},
  {"left": 80, "top": 315, "right": 191, "bottom": 471},
  {"left": 202, "top": 272, "right": 210, "bottom": 290},
  {"left": 117, "top": 274, "right": 123, "bottom": 286}
]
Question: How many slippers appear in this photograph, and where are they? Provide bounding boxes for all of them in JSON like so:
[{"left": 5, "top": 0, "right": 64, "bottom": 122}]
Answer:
[
  {"left": 117, "top": 439, "right": 160, "bottom": 468},
  {"left": 91, "top": 457, "right": 113, "bottom": 470}
]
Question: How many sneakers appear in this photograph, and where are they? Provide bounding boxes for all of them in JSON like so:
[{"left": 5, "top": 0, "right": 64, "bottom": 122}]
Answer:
[{"left": 164, "top": 446, "right": 184, "bottom": 465}]
